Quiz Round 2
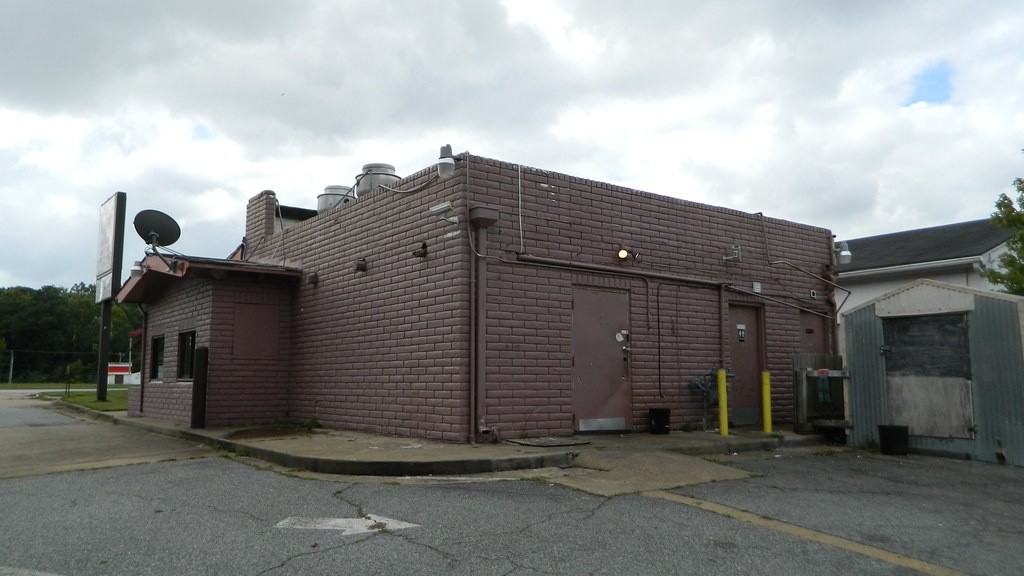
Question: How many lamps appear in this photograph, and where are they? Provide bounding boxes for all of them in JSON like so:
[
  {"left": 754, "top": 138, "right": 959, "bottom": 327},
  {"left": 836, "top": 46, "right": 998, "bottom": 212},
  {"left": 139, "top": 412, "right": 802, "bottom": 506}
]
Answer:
[
  {"left": 832, "top": 241, "right": 852, "bottom": 265},
  {"left": 618, "top": 244, "right": 639, "bottom": 260},
  {"left": 437, "top": 145, "right": 465, "bottom": 178},
  {"left": 130, "top": 261, "right": 147, "bottom": 279}
]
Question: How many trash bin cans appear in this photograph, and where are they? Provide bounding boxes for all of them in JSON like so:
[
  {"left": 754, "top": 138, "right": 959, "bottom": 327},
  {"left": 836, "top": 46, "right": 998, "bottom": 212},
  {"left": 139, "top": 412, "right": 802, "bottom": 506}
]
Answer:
[
  {"left": 878, "top": 424, "right": 908, "bottom": 456},
  {"left": 647, "top": 407, "right": 671, "bottom": 435}
]
